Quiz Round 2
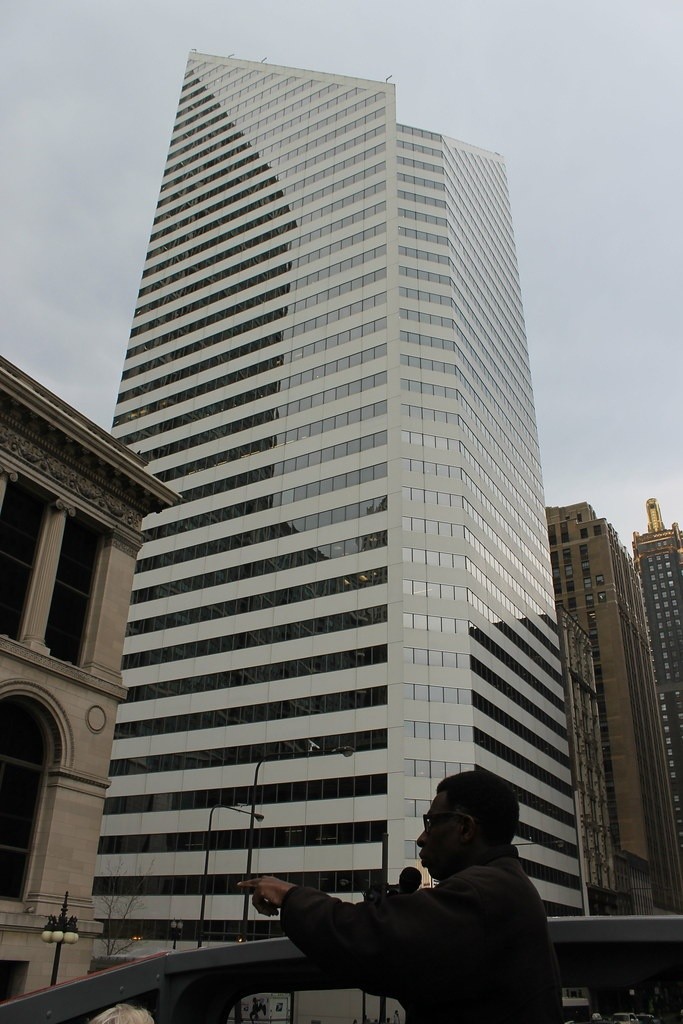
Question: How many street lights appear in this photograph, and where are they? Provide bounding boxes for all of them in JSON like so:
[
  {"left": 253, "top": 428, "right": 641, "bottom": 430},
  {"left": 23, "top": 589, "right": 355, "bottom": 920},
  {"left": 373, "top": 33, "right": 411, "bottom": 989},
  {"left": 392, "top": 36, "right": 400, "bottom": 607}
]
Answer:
[
  {"left": 195, "top": 805, "right": 265, "bottom": 950},
  {"left": 629, "top": 887, "right": 674, "bottom": 914},
  {"left": 169, "top": 918, "right": 184, "bottom": 953},
  {"left": 238, "top": 746, "right": 356, "bottom": 939},
  {"left": 42, "top": 894, "right": 78, "bottom": 988}
]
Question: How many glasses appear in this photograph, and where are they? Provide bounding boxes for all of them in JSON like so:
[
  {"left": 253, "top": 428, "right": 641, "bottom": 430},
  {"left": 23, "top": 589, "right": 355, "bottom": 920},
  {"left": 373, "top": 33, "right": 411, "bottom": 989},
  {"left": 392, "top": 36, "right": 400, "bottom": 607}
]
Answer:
[{"left": 423, "top": 811, "right": 482, "bottom": 833}]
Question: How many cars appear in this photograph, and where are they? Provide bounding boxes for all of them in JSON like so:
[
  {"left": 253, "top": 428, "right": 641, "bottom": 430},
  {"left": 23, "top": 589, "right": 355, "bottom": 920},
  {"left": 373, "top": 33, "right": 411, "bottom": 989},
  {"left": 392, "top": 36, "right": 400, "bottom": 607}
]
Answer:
[{"left": 565, "top": 1012, "right": 664, "bottom": 1024}]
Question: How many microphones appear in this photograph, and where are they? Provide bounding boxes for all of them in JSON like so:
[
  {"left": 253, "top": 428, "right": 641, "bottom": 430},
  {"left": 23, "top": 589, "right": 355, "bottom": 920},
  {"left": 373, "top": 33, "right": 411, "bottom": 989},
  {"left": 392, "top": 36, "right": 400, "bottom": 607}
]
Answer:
[{"left": 398, "top": 866, "right": 421, "bottom": 894}]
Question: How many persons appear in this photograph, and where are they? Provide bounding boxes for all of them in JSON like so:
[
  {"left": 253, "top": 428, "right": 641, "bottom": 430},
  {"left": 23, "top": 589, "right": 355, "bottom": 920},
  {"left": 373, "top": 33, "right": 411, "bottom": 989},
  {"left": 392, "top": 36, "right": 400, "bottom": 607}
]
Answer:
[
  {"left": 238, "top": 770, "right": 566, "bottom": 1024},
  {"left": 397, "top": 867, "right": 422, "bottom": 895}
]
